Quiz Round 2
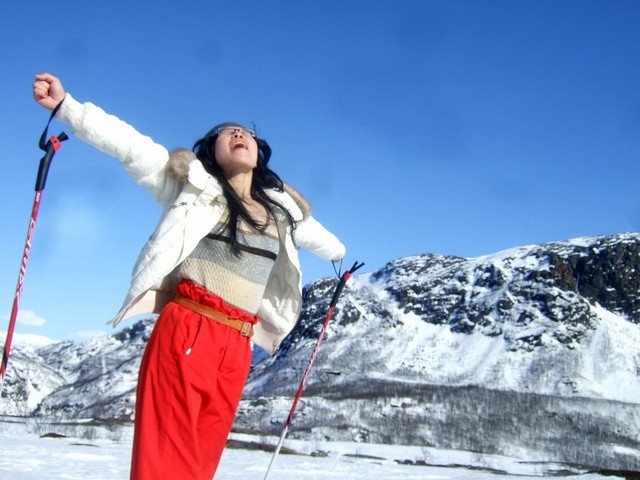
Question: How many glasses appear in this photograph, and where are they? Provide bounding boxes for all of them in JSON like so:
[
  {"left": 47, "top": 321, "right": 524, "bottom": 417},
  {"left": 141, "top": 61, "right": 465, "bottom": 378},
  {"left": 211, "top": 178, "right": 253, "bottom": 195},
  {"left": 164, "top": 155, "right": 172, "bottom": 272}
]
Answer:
[{"left": 214, "top": 127, "right": 256, "bottom": 137}]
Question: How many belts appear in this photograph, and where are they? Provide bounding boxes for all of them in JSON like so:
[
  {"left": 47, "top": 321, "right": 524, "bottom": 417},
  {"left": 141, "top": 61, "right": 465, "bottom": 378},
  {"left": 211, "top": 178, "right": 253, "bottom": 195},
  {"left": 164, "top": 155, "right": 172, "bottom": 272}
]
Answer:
[{"left": 174, "top": 296, "right": 254, "bottom": 338}]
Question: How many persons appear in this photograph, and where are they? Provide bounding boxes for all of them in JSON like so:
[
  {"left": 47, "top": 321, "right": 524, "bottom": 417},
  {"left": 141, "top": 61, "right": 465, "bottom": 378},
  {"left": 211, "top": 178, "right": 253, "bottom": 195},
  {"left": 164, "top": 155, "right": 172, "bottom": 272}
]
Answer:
[{"left": 32, "top": 72, "right": 347, "bottom": 480}]
